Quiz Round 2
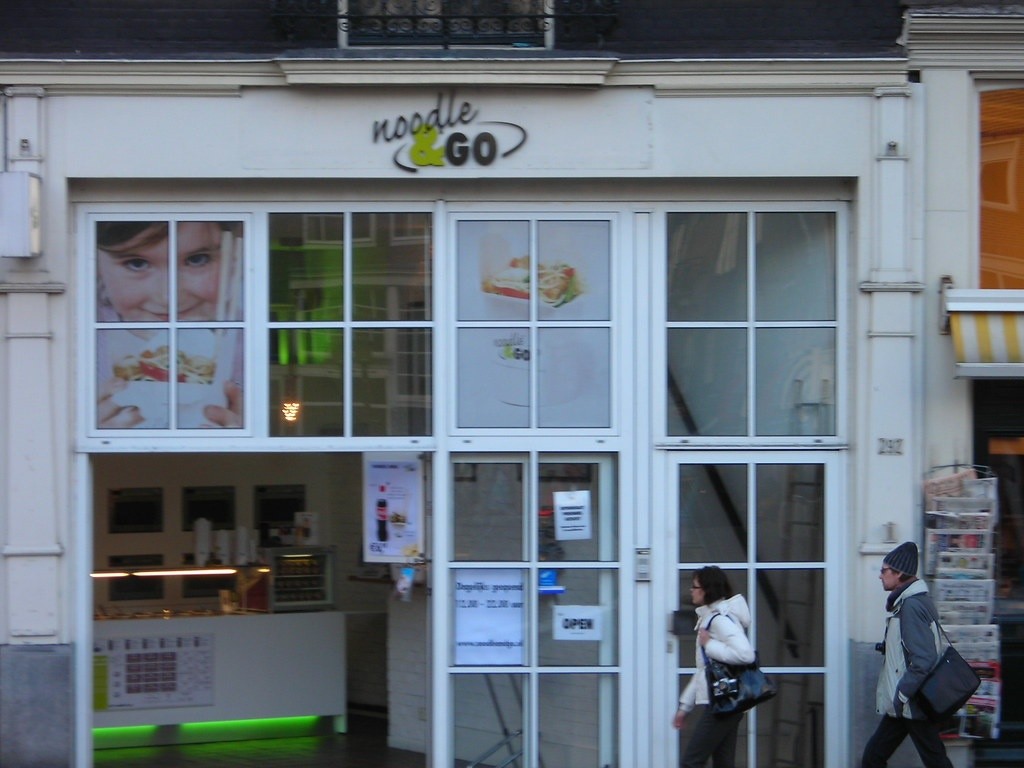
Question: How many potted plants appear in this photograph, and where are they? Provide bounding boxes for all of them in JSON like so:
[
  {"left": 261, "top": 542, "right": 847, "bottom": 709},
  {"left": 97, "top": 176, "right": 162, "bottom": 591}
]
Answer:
[{"left": 538, "top": 538, "right": 566, "bottom": 585}]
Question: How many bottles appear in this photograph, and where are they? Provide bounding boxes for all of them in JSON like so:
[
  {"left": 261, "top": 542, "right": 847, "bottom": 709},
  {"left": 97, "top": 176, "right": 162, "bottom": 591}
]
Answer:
[{"left": 375, "top": 485, "right": 389, "bottom": 542}]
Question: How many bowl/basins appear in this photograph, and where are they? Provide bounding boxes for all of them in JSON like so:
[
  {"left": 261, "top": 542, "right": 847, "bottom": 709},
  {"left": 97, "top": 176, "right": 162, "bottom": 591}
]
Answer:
[
  {"left": 110, "top": 357, "right": 220, "bottom": 411},
  {"left": 481, "top": 285, "right": 596, "bottom": 320}
]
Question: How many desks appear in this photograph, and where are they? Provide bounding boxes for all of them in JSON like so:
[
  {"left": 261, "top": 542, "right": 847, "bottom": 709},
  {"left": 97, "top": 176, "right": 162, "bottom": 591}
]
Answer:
[{"left": 347, "top": 575, "right": 566, "bottom": 592}]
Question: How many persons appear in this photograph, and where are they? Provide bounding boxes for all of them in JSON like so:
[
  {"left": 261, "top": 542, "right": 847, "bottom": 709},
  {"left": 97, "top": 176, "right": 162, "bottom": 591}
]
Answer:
[
  {"left": 673, "top": 565, "right": 755, "bottom": 768},
  {"left": 95, "top": 221, "right": 245, "bottom": 428},
  {"left": 860, "top": 543, "right": 955, "bottom": 768}
]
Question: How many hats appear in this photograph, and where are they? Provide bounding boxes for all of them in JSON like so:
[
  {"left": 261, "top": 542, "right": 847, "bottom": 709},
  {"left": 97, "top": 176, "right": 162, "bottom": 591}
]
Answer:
[{"left": 883, "top": 541, "right": 918, "bottom": 576}]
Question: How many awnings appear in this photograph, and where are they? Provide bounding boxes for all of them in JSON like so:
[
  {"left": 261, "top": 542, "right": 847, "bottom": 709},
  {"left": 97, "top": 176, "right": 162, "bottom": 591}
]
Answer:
[{"left": 939, "top": 275, "right": 1024, "bottom": 381}]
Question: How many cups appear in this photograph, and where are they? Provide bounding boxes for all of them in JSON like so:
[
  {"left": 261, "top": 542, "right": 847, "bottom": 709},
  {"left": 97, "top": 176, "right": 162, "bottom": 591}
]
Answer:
[{"left": 193, "top": 519, "right": 260, "bottom": 567}]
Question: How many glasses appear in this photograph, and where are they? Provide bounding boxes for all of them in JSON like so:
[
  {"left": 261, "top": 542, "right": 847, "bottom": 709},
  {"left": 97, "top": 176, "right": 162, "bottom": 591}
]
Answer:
[
  {"left": 880, "top": 567, "right": 891, "bottom": 574},
  {"left": 691, "top": 583, "right": 702, "bottom": 589}
]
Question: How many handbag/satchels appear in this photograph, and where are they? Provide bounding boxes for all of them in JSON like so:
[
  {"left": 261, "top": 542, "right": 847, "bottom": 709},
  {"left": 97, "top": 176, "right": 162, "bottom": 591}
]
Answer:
[
  {"left": 700, "top": 614, "right": 777, "bottom": 720},
  {"left": 913, "top": 615, "right": 981, "bottom": 725}
]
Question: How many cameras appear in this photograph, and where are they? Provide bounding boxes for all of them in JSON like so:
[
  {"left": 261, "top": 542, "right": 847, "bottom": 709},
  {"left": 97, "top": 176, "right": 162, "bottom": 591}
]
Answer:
[{"left": 875, "top": 640, "right": 886, "bottom": 655}]
